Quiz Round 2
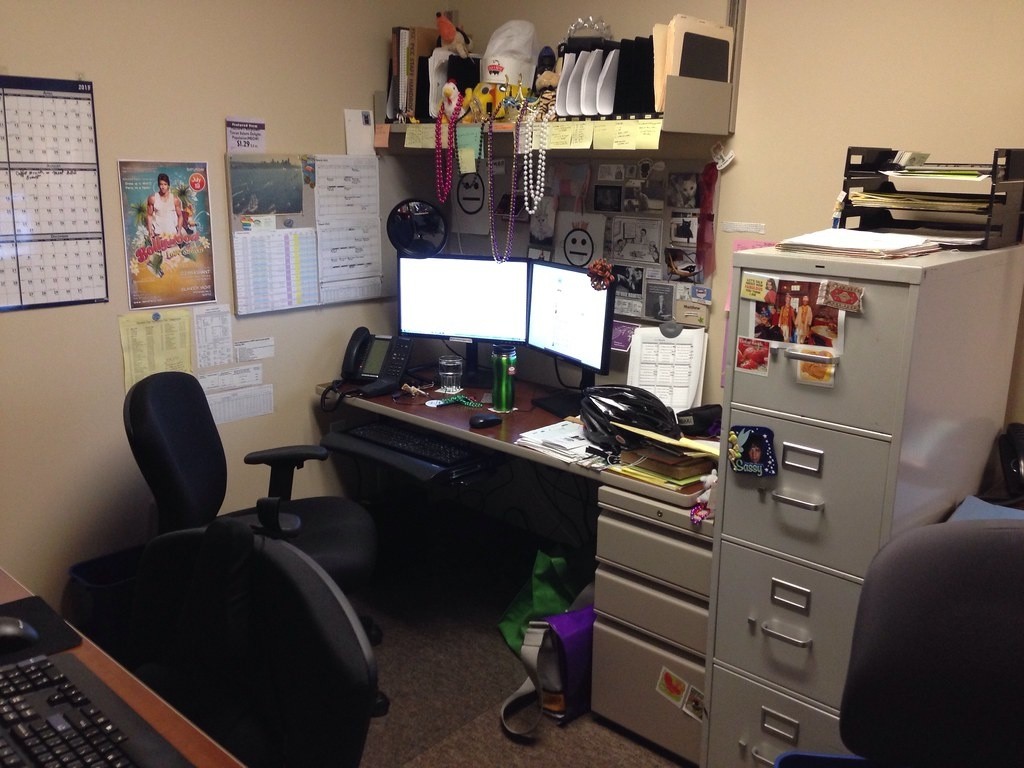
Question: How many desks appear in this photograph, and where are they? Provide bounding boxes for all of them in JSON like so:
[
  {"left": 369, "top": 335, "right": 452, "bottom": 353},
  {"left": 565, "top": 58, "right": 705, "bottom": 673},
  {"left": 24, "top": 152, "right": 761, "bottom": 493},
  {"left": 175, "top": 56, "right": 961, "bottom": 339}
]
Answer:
[
  {"left": 0, "top": 568, "right": 245, "bottom": 768},
  {"left": 316, "top": 370, "right": 719, "bottom": 763}
]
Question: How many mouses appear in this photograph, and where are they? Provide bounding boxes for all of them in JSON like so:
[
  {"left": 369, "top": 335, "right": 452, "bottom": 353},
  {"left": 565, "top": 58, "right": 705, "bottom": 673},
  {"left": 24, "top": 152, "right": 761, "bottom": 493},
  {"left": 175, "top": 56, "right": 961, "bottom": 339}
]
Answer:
[
  {"left": 469, "top": 414, "right": 502, "bottom": 428},
  {"left": 0, "top": 616, "right": 38, "bottom": 651}
]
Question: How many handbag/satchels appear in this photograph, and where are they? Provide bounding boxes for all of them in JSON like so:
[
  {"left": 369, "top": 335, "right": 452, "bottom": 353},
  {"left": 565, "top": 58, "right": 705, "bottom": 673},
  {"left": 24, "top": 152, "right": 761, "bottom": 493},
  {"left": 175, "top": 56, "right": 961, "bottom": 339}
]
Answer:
[
  {"left": 501, "top": 602, "right": 596, "bottom": 735},
  {"left": 496, "top": 548, "right": 577, "bottom": 658}
]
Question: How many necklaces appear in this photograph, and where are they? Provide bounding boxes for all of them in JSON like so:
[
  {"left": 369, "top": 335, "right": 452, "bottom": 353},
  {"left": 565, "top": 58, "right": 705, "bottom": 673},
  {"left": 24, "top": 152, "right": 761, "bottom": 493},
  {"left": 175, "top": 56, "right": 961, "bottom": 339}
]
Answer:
[
  {"left": 435, "top": 94, "right": 488, "bottom": 202},
  {"left": 524, "top": 107, "right": 555, "bottom": 215},
  {"left": 487, "top": 100, "right": 527, "bottom": 263},
  {"left": 442, "top": 395, "right": 482, "bottom": 407}
]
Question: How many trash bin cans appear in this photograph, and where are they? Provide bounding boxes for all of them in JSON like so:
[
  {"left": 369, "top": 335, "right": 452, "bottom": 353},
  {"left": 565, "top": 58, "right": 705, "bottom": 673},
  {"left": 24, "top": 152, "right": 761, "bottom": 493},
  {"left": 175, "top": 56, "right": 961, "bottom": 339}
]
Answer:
[{"left": 67, "top": 544, "right": 147, "bottom": 671}]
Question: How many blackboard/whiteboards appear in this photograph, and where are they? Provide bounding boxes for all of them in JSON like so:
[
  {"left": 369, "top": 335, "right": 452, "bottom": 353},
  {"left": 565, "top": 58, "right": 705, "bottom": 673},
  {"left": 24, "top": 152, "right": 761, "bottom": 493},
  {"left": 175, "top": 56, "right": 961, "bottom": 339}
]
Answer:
[{"left": 222, "top": 151, "right": 455, "bottom": 317}]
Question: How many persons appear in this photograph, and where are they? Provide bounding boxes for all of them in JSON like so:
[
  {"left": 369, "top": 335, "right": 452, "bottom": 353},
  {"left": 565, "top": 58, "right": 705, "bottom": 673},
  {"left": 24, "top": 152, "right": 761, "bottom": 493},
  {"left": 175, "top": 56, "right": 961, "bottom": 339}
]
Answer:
[
  {"left": 755, "top": 294, "right": 812, "bottom": 343},
  {"left": 654, "top": 295, "right": 666, "bottom": 317},
  {"left": 146, "top": 173, "right": 182, "bottom": 240},
  {"left": 747, "top": 437, "right": 762, "bottom": 463},
  {"left": 764, "top": 278, "right": 776, "bottom": 303}
]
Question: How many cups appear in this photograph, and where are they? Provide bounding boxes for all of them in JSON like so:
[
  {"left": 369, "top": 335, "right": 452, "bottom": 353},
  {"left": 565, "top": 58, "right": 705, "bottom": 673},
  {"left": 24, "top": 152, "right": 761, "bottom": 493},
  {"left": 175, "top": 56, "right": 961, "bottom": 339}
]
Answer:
[{"left": 438, "top": 355, "right": 463, "bottom": 392}]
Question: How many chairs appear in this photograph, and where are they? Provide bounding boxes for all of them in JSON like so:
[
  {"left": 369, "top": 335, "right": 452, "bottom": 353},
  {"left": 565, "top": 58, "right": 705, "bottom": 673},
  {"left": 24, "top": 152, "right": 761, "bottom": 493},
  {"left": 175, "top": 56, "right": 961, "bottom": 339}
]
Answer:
[
  {"left": 121, "top": 517, "right": 390, "bottom": 768},
  {"left": 123, "top": 371, "right": 390, "bottom": 715}
]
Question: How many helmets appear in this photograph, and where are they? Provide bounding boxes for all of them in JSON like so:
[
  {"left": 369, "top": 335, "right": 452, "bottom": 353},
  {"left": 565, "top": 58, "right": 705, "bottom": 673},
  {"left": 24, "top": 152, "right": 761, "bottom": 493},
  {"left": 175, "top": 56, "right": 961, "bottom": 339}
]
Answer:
[{"left": 581, "top": 384, "right": 683, "bottom": 463}]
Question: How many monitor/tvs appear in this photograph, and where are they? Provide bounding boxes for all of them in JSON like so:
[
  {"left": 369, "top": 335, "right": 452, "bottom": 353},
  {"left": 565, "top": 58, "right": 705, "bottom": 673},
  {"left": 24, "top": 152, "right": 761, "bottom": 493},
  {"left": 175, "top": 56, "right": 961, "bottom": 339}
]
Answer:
[
  {"left": 398, "top": 253, "right": 531, "bottom": 389},
  {"left": 529, "top": 259, "right": 616, "bottom": 418}
]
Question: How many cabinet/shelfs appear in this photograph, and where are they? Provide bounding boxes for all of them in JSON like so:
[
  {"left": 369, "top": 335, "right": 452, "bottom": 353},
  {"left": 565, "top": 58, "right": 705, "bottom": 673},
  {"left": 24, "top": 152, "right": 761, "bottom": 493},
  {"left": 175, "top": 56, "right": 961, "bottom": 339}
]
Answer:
[{"left": 699, "top": 244, "right": 1024, "bottom": 768}]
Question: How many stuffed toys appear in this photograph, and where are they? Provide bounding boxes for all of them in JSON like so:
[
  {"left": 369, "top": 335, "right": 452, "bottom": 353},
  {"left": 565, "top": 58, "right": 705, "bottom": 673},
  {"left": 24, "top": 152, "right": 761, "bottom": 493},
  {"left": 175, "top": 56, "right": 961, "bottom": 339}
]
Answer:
[
  {"left": 436, "top": 12, "right": 474, "bottom": 59},
  {"left": 462, "top": 82, "right": 529, "bottom": 120},
  {"left": 441, "top": 80, "right": 467, "bottom": 117},
  {"left": 529, "top": 71, "right": 560, "bottom": 120}
]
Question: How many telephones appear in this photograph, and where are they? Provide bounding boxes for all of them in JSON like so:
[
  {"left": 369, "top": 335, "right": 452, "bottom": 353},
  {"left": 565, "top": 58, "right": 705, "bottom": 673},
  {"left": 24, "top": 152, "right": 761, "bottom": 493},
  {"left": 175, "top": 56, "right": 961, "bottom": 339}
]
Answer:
[{"left": 340, "top": 326, "right": 415, "bottom": 386}]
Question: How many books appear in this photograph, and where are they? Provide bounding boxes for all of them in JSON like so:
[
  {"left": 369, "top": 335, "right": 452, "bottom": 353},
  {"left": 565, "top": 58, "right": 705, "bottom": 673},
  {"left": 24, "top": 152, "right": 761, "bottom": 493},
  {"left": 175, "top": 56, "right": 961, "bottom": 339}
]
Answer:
[{"left": 620, "top": 451, "right": 715, "bottom": 480}]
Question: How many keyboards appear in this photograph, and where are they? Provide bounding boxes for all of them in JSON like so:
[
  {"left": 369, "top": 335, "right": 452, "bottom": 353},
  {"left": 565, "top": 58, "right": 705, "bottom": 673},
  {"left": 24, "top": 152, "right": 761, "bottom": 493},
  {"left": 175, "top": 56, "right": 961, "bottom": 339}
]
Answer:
[
  {"left": 0, "top": 654, "right": 196, "bottom": 768},
  {"left": 348, "top": 425, "right": 479, "bottom": 467}
]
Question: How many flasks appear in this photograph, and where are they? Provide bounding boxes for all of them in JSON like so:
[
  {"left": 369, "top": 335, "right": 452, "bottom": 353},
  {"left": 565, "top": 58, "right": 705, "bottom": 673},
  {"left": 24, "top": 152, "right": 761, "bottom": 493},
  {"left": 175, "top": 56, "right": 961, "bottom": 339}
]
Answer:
[{"left": 491, "top": 344, "right": 518, "bottom": 411}]
